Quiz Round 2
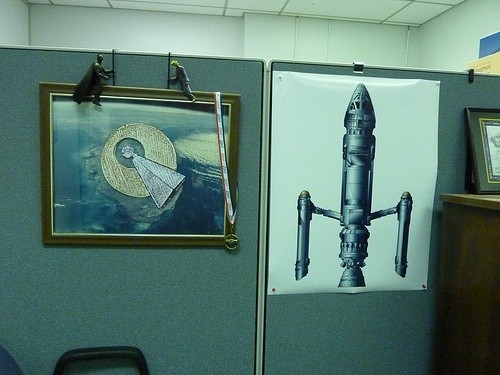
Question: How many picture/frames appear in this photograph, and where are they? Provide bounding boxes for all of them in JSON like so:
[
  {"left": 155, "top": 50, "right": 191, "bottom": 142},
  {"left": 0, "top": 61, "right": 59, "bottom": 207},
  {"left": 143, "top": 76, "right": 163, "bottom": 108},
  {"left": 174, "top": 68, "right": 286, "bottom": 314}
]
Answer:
[
  {"left": 38, "top": 81, "right": 242, "bottom": 251},
  {"left": 463, "top": 105, "right": 500, "bottom": 195}
]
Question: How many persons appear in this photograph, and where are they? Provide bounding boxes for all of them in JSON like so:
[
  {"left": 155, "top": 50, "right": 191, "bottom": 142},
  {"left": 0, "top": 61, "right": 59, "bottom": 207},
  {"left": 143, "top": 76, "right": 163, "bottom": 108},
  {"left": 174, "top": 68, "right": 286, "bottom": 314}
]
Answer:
[
  {"left": 73, "top": 55, "right": 115, "bottom": 106},
  {"left": 169, "top": 61, "right": 196, "bottom": 103}
]
